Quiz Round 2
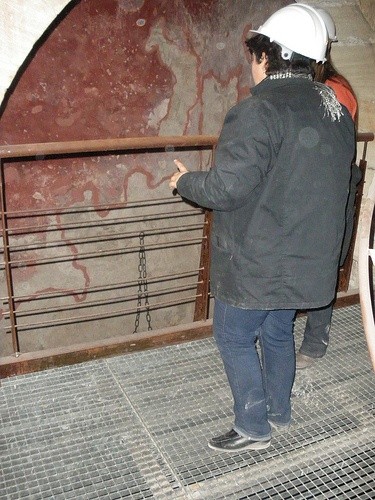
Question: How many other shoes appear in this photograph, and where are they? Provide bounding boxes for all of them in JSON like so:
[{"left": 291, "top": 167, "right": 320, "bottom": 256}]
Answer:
[{"left": 296, "top": 352, "right": 318, "bottom": 370}]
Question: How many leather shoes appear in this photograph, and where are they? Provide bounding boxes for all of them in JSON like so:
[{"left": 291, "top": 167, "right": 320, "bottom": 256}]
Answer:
[
  {"left": 269, "top": 420, "right": 290, "bottom": 431},
  {"left": 207, "top": 429, "right": 271, "bottom": 452}
]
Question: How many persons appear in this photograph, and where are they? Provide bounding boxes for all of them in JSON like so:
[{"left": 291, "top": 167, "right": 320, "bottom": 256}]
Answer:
[
  {"left": 169, "top": 3, "right": 362, "bottom": 452},
  {"left": 295, "top": 9, "right": 357, "bottom": 369}
]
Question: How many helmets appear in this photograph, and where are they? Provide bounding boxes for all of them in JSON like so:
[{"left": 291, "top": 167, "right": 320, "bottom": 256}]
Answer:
[
  {"left": 249, "top": 3, "right": 327, "bottom": 65},
  {"left": 315, "top": 8, "right": 338, "bottom": 42}
]
